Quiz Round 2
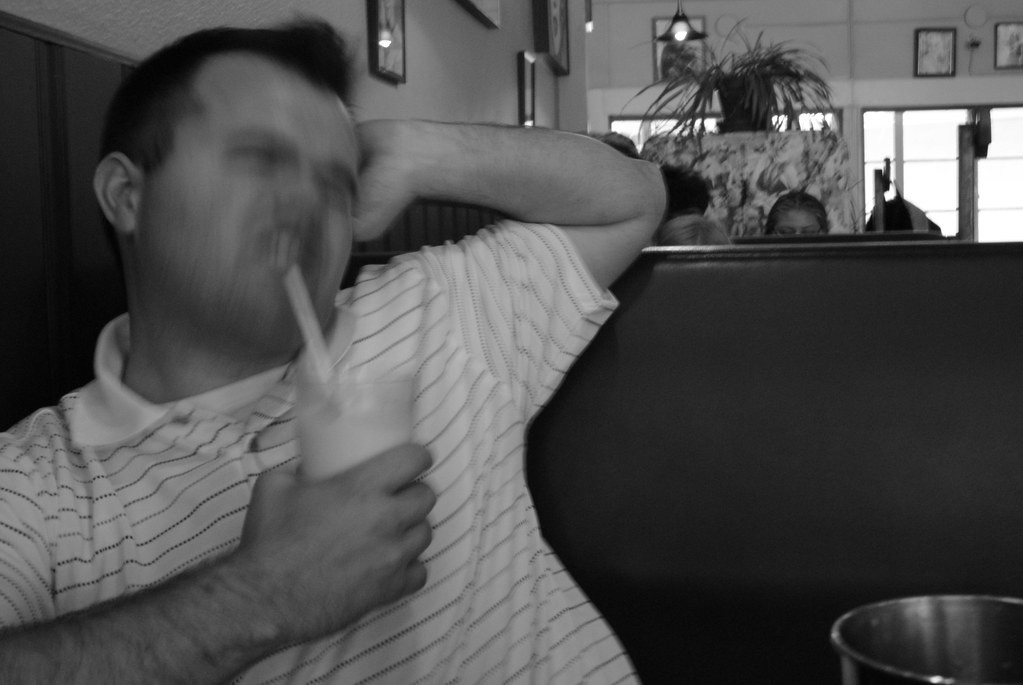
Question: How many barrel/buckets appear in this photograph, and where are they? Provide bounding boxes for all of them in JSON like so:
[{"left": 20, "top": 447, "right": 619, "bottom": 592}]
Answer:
[{"left": 829, "top": 595, "right": 1023, "bottom": 685}]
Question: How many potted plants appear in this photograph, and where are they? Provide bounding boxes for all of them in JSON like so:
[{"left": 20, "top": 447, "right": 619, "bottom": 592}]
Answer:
[{"left": 617, "top": 14, "right": 841, "bottom": 154}]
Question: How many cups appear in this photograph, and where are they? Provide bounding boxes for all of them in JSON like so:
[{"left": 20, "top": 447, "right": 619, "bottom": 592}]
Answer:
[{"left": 300, "top": 374, "right": 417, "bottom": 489}]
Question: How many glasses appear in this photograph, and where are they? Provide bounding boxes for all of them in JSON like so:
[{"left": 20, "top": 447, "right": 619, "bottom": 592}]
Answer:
[{"left": 772, "top": 226, "right": 822, "bottom": 236}]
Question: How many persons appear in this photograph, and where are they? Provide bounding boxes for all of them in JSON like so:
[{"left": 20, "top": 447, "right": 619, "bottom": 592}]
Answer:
[
  {"left": 0, "top": 21, "right": 671, "bottom": 685},
  {"left": 573, "top": 144, "right": 828, "bottom": 245}
]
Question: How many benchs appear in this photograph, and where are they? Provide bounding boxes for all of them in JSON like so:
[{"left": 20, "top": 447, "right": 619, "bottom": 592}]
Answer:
[{"left": 296, "top": 234, "right": 1023, "bottom": 685}]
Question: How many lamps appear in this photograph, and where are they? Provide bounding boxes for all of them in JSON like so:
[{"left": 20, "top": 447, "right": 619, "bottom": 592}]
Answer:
[{"left": 655, "top": 0, "right": 709, "bottom": 44}]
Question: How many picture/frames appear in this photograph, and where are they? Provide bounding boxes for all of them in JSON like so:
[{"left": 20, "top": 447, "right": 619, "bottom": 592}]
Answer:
[
  {"left": 365, "top": 0, "right": 707, "bottom": 130},
  {"left": 914, "top": 27, "right": 956, "bottom": 77},
  {"left": 994, "top": 21, "right": 1023, "bottom": 70}
]
